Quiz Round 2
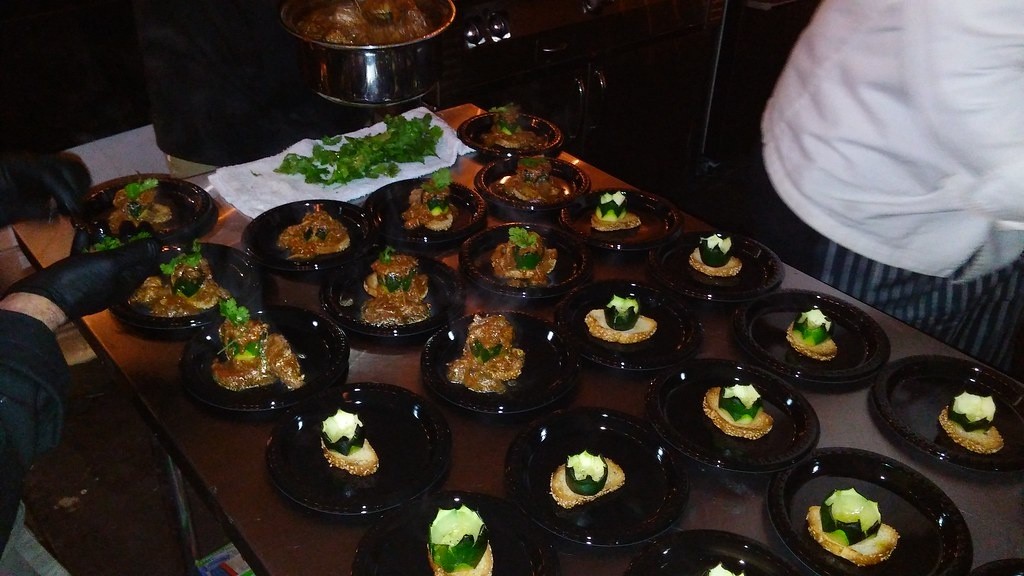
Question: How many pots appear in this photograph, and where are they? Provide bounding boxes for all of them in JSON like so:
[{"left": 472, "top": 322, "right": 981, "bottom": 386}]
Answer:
[{"left": 277, "top": 0, "right": 457, "bottom": 104}]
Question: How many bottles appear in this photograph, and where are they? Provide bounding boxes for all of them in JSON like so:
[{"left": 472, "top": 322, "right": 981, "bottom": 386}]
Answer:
[
  {"left": 564, "top": 75, "right": 585, "bottom": 139},
  {"left": 587, "top": 64, "right": 608, "bottom": 128}
]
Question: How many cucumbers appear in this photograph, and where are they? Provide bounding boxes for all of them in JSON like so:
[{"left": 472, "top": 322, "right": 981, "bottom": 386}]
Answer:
[
  {"left": 604, "top": 234, "right": 996, "bottom": 576},
  {"left": 428, "top": 503, "right": 488, "bottom": 572},
  {"left": 596, "top": 191, "right": 627, "bottom": 222},
  {"left": 322, "top": 408, "right": 365, "bottom": 455},
  {"left": 565, "top": 450, "right": 608, "bottom": 496}
]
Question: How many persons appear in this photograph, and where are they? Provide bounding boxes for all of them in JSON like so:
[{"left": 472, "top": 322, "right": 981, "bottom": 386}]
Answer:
[
  {"left": 761, "top": 0, "right": 1024, "bottom": 387},
  {"left": 0, "top": 149, "right": 160, "bottom": 567}
]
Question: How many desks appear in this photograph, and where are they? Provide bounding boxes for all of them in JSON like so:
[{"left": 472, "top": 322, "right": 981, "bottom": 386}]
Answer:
[{"left": 12, "top": 105, "right": 1024, "bottom": 576}]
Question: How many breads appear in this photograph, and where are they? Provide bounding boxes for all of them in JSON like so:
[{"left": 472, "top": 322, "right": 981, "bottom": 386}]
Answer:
[
  {"left": 807, "top": 505, "right": 899, "bottom": 565},
  {"left": 550, "top": 458, "right": 625, "bottom": 509},
  {"left": 939, "top": 405, "right": 1004, "bottom": 453},
  {"left": 427, "top": 544, "right": 493, "bottom": 576},
  {"left": 704, "top": 387, "right": 773, "bottom": 440},
  {"left": 585, "top": 309, "right": 657, "bottom": 343},
  {"left": 591, "top": 212, "right": 641, "bottom": 231},
  {"left": 689, "top": 247, "right": 743, "bottom": 276},
  {"left": 787, "top": 321, "right": 837, "bottom": 361},
  {"left": 321, "top": 437, "right": 379, "bottom": 476}
]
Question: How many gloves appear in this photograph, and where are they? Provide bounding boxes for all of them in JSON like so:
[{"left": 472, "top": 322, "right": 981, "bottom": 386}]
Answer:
[
  {"left": 7, "top": 151, "right": 92, "bottom": 216},
  {"left": 4, "top": 221, "right": 163, "bottom": 321}
]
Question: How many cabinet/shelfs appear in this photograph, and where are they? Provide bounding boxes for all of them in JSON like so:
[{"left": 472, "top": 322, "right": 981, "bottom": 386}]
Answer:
[{"left": 451, "top": 1, "right": 725, "bottom": 194}]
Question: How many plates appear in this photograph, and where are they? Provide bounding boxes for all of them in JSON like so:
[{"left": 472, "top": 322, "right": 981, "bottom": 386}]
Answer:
[
  {"left": 552, "top": 279, "right": 703, "bottom": 370},
  {"left": 505, "top": 406, "right": 690, "bottom": 547},
  {"left": 240, "top": 200, "right": 375, "bottom": 271},
  {"left": 871, "top": 354, "right": 1024, "bottom": 472},
  {"left": 364, "top": 178, "right": 487, "bottom": 244},
  {"left": 352, "top": 491, "right": 562, "bottom": 576},
  {"left": 474, "top": 155, "right": 589, "bottom": 211},
  {"left": 421, "top": 310, "right": 579, "bottom": 414},
  {"left": 648, "top": 231, "right": 785, "bottom": 302},
  {"left": 73, "top": 173, "right": 219, "bottom": 245},
  {"left": 645, "top": 359, "right": 820, "bottom": 473},
  {"left": 177, "top": 305, "right": 350, "bottom": 411},
  {"left": 971, "top": 558, "right": 1024, "bottom": 576},
  {"left": 558, "top": 188, "right": 684, "bottom": 250},
  {"left": 622, "top": 529, "right": 805, "bottom": 576},
  {"left": 319, "top": 246, "right": 466, "bottom": 337},
  {"left": 266, "top": 382, "right": 452, "bottom": 515},
  {"left": 732, "top": 288, "right": 891, "bottom": 383},
  {"left": 459, "top": 222, "right": 592, "bottom": 298},
  {"left": 107, "top": 242, "right": 259, "bottom": 338},
  {"left": 457, "top": 112, "right": 564, "bottom": 156},
  {"left": 766, "top": 447, "right": 973, "bottom": 576}
]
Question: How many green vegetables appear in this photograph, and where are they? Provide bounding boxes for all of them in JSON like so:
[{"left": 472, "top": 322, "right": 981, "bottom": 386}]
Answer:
[
  {"left": 219, "top": 297, "right": 249, "bottom": 327},
  {"left": 274, "top": 112, "right": 443, "bottom": 183},
  {"left": 420, "top": 169, "right": 452, "bottom": 192},
  {"left": 124, "top": 178, "right": 158, "bottom": 198},
  {"left": 159, "top": 240, "right": 203, "bottom": 275},
  {"left": 508, "top": 227, "right": 535, "bottom": 248},
  {"left": 83, "top": 232, "right": 153, "bottom": 251}
]
